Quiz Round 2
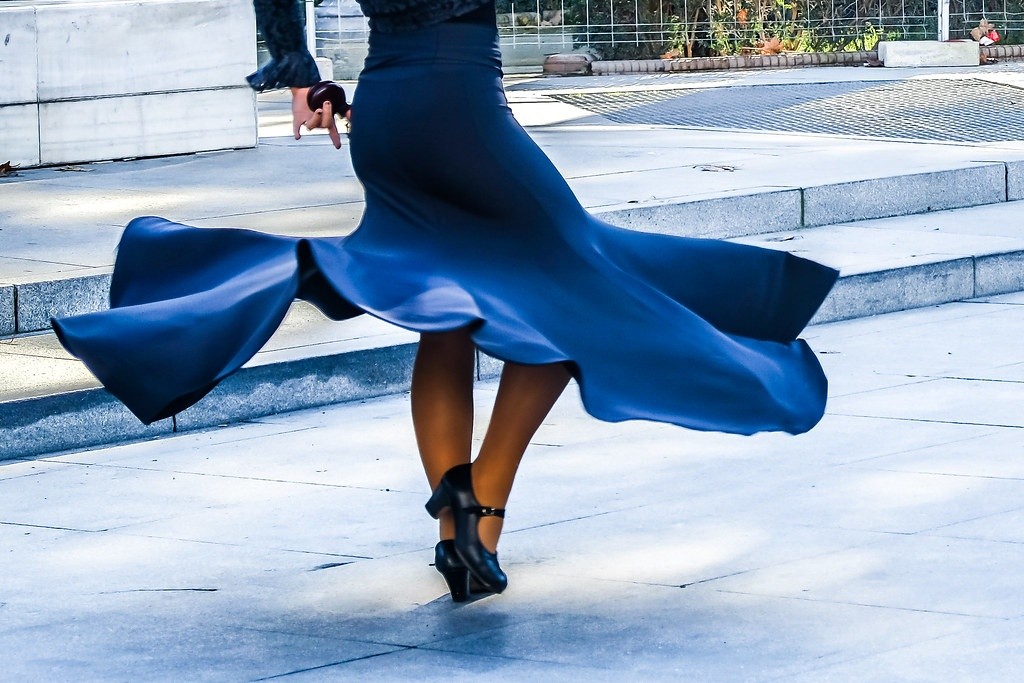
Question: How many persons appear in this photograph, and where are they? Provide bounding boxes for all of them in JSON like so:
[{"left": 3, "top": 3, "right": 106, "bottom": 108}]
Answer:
[{"left": 49, "top": 0, "right": 841, "bottom": 601}]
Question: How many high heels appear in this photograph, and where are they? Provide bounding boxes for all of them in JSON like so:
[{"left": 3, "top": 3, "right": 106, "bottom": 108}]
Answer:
[
  {"left": 427, "top": 539, "right": 480, "bottom": 602},
  {"left": 425, "top": 462, "right": 507, "bottom": 594}
]
